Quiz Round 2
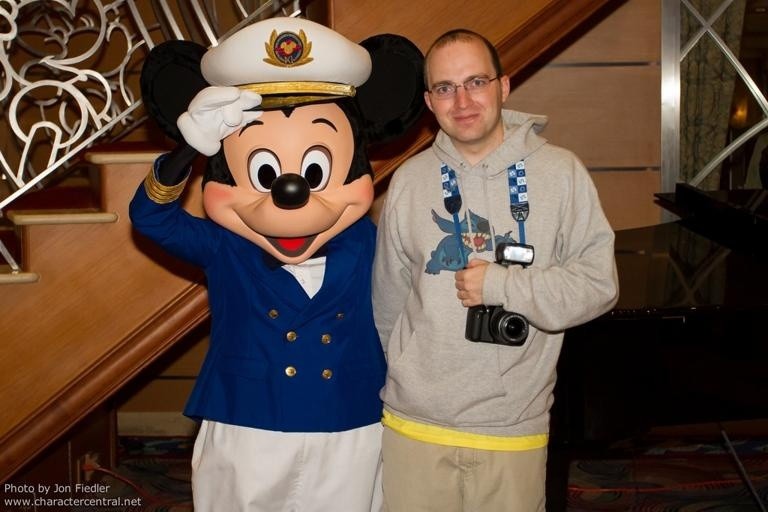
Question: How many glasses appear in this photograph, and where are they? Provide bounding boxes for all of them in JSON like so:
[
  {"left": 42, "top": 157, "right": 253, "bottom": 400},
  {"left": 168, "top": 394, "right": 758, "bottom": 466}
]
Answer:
[{"left": 428, "top": 77, "right": 497, "bottom": 97}]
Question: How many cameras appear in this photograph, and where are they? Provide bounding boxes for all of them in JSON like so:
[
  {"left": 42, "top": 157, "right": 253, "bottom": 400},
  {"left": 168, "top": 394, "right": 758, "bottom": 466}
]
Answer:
[{"left": 465, "top": 243, "right": 534, "bottom": 345}]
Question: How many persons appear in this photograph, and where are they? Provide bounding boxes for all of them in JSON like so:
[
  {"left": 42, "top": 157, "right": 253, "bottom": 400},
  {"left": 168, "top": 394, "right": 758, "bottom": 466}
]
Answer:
[{"left": 371, "top": 29, "right": 619, "bottom": 512}]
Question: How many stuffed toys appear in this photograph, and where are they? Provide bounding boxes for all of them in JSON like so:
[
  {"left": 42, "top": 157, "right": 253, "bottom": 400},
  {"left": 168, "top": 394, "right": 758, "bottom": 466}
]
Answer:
[{"left": 129, "top": 17, "right": 424, "bottom": 512}]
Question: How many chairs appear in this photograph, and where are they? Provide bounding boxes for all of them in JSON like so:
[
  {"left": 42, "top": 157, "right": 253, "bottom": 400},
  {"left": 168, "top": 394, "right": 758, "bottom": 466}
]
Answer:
[
  {"left": 155, "top": 335, "right": 211, "bottom": 376},
  {"left": 106, "top": 381, "right": 209, "bottom": 467}
]
